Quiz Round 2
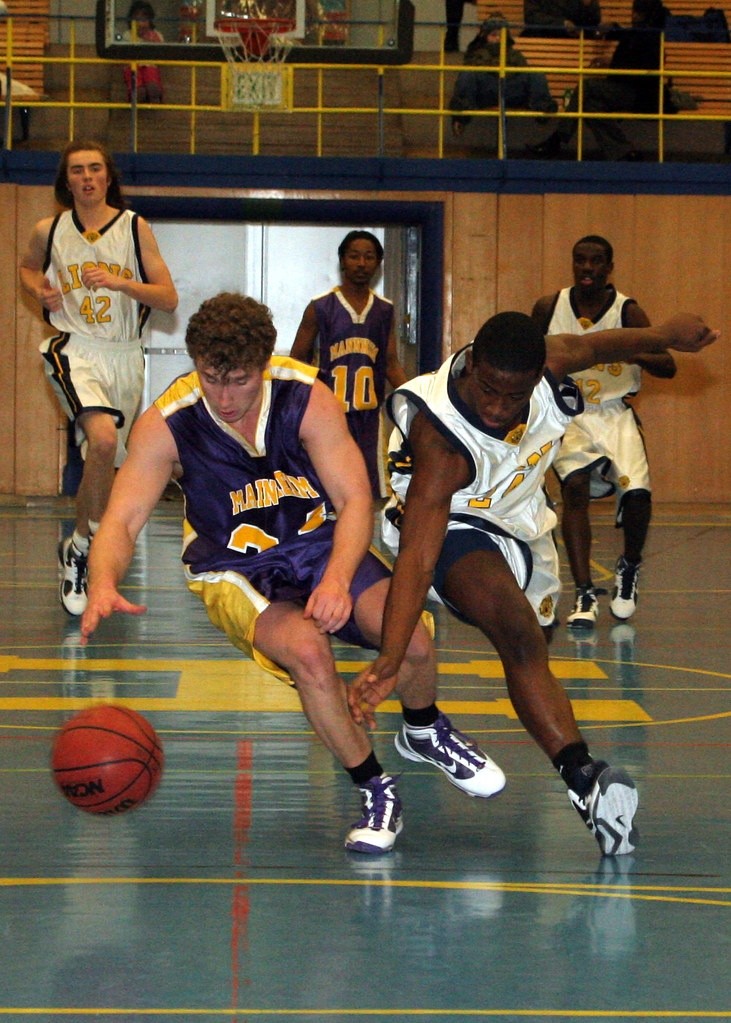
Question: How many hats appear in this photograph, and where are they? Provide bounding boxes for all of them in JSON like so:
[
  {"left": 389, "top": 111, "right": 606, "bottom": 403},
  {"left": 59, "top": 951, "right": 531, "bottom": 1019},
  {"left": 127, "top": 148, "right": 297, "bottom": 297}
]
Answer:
[{"left": 480, "top": 12, "right": 515, "bottom": 46}]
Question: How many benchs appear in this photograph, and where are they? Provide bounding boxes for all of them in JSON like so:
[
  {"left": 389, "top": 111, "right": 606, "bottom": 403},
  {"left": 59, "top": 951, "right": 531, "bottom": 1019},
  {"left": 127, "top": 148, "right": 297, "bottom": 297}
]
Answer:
[
  {"left": 0, "top": 0, "right": 50, "bottom": 143},
  {"left": 477, "top": 0, "right": 731, "bottom": 155}
]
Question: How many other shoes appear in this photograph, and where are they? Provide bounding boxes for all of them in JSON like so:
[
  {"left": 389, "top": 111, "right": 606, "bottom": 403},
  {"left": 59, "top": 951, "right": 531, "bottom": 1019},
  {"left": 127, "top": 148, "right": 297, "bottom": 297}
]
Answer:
[
  {"left": 449, "top": 96, "right": 469, "bottom": 126},
  {"left": 136, "top": 82, "right": 160, "bottom": 104},
  {"left": 535, "top": 94, "right": 558, "bottom": 124},
  {"left": 533, "top": 137, "right": 561, "bottom": 159}
]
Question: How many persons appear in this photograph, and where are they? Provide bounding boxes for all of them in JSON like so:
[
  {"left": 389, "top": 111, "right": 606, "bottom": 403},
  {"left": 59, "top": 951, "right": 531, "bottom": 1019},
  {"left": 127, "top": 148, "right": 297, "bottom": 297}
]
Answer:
[
  {"left": 287, "top": 231, "right": 407, "bottom": 503},
  {"left": 447, "top": 11, "right": 559, "bottom": 111},
  {"left": 119, "top": 0, "right": 164, "bottom": 104},
  {"left": 347, "top": 312, "right": 721, "bottom": 855},
  {"left": 18, "top": 140, "right": 178, "bottom": 617},
  {"left": 522, "top": 0, "right": 728, "bottom": 39},
  {"left": 79, "top": 291, "right": 507, "bottom": 854},
  {"left": 528, "top": 235, "right": 677, "bottom": 626},
  {"left": 546, "top": 35, "right": 696, "bottom": 161}
]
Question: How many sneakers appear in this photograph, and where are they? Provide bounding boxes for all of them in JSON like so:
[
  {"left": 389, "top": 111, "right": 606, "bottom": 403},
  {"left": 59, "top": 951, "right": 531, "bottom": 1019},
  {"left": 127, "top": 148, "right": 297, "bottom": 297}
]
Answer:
[
  {"left": 395, "top": 711, "right": 506, "bottom": 798},
  {"left": 60, "top": 633, "right": 87, "bottom": 698},
  {"left": 588, "top": 853, "right": 635, "bottom": 954},
  {"left": 568, "top": 760, "right": 640, "bottom": 856},
  {"left": 609, "top": 555, "right": 640, "bottom": 620},
  {"left": 566, "top": 628, "right": 598, "bottom": 661},
  {"left": 610, "top": 623, "right": 640, "bottom": 678},
  {"left": 567, "top": 585, "right": 607, "bottom": 629},
  {"left": 57, "top": 526, "right": 94, "bottom": 616},
  {"left": 90, "top": 675, "right": 114, "bottom": 699},
  {"left": 348, "top": 851, "right": 403, "bottom": 907},
  {"left": 344, "top": 769, "right": 404, "bottom": 853}
]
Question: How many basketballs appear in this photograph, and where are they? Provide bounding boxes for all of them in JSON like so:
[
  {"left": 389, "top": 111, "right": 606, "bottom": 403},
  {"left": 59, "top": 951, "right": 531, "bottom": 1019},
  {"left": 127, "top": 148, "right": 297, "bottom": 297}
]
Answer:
[{"left": 51, "top": 702, "right": 167, "bottom": 819}]
